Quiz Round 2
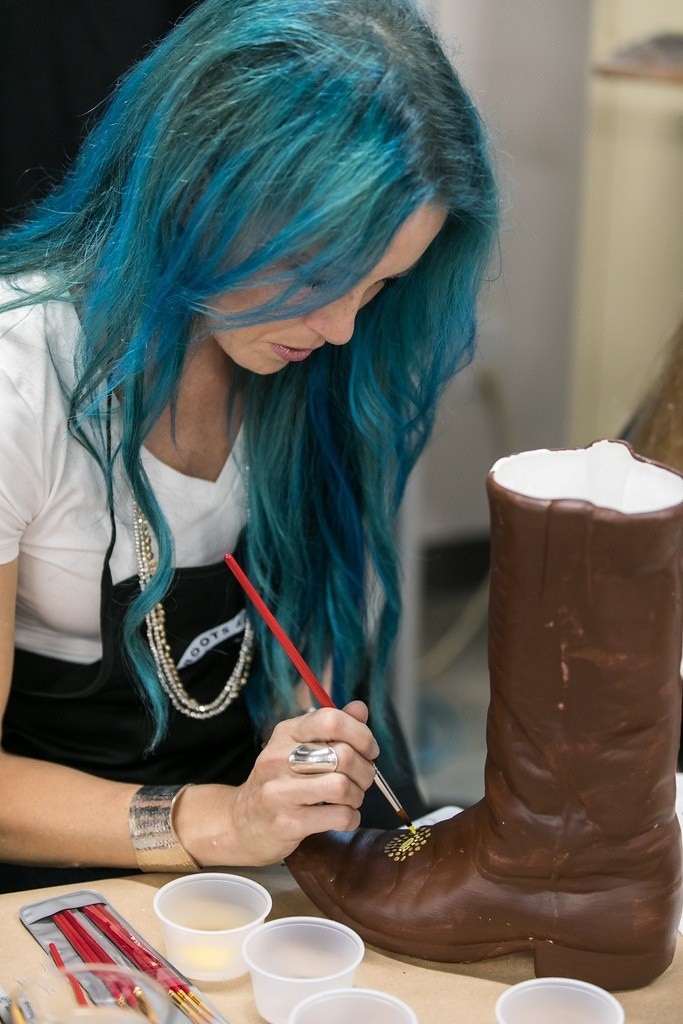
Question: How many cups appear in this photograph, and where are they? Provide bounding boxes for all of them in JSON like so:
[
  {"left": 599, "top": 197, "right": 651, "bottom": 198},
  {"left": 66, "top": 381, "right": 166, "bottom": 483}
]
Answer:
[{"left": 36, "top": 963, "right": 171, "bottom": 1024}]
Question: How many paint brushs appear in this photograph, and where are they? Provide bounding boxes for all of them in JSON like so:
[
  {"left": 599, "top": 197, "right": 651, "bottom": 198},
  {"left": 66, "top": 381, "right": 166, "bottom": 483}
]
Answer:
[
  {"left": 49, "top": 902, "right": 223, "bottom": 1024},
  {"left": 224, "top": 553, "right": 419, "bottom": 837}
]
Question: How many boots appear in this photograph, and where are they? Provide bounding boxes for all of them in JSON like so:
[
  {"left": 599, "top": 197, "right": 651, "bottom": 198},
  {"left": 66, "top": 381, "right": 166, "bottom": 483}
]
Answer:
[{"left": 283, "top": 438, "right": 683, "bottom": 992}]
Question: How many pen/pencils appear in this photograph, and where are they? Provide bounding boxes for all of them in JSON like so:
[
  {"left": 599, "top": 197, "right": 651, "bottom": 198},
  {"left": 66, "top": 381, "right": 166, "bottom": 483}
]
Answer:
[{"left": 10, "top": 998, "right": 26, "bottom": 1024}]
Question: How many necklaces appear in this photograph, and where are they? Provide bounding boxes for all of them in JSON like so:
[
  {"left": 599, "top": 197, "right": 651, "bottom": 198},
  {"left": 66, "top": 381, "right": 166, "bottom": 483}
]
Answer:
[{"left": 129, "top": 448, "right": 266, "bottom": 720}]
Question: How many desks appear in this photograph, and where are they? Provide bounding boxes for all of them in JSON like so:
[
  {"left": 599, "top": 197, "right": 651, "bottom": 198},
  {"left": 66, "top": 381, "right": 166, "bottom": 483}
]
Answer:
[{"left": 0, "top": 861, "right": 683, "bottom": 1024}]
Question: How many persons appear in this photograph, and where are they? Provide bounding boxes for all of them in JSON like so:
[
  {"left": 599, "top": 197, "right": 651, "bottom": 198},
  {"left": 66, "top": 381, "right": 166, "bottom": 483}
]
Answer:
[{"left": 0, "top": 0, "right": 500, "bottom": 883}]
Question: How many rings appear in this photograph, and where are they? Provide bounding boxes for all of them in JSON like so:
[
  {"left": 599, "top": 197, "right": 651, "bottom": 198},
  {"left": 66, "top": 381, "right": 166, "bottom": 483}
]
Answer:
[{"left": 288, "top": 741, "right": 341, "bottom": 776}]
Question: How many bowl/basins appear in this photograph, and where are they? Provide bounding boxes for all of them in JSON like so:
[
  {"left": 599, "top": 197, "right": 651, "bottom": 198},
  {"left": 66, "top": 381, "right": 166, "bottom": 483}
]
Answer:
[
  {"left": 242, "top": 916, "right": 363, "bottom": 1024},
  {"left": 152, "top": 873, "right": 272, "bottom": 983},
  {"left": 495, "top": 977, "right": 625, "bottom": 1024},
  {"left": 288, "top": 988, "right": 418, "bottom": 1024}
]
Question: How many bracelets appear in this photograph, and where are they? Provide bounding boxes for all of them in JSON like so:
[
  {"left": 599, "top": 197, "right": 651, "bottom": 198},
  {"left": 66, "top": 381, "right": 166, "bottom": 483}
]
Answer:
[{"left": 128, "top": 783, "right": 202, "bottom": 873}]
